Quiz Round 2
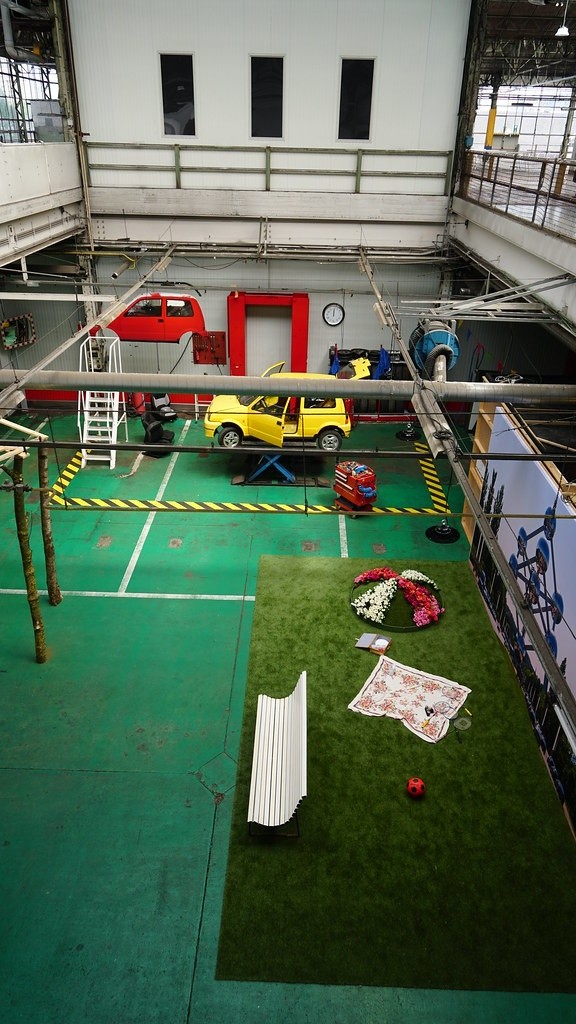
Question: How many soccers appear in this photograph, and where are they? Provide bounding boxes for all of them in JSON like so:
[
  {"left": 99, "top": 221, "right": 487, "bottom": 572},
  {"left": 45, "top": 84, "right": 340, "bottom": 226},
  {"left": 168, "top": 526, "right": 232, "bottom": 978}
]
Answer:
[{"left": 406, "top": 777, "right": 426, "bottom": 797}]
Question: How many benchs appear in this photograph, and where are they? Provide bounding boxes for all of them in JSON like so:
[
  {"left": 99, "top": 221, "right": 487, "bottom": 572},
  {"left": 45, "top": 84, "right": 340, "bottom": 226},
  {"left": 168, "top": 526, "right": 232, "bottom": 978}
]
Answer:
[{"left": 247, "top": 669, "right": 308, "bottom": 845}]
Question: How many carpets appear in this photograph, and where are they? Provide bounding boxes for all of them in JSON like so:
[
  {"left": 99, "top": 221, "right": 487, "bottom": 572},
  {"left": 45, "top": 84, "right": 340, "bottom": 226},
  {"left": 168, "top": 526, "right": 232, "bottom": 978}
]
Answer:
[
  {"left": 345, "top": 652, "right": 471, "bottom": 743},
  {"left": 211, "top": 554, "right": 576, "bottom": 995}
]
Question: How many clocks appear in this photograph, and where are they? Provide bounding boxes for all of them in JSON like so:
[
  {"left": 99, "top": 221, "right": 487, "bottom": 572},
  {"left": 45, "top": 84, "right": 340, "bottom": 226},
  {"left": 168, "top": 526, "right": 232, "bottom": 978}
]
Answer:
[{"left": 322, "top": 303, "right": 346, "bottom": 326}]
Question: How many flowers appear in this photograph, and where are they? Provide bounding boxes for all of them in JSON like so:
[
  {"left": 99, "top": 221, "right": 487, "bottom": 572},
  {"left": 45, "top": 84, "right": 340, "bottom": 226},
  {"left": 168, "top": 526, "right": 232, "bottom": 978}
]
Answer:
[{"left": 352, "top": 567, "right": 446, "bottom": 628}]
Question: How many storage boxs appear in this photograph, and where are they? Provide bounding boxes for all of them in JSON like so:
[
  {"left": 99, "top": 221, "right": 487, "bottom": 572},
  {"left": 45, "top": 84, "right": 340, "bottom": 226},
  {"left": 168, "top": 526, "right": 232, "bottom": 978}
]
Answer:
[{"left": 354, "top": 631, "right": 391, "bottom": 656}]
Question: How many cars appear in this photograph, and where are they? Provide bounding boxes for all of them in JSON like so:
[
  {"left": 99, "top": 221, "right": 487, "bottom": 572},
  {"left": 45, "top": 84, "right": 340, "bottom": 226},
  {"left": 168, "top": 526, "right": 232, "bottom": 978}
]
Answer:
[{"left": 206, "top": 360, "right": 366, "bottom": 459}]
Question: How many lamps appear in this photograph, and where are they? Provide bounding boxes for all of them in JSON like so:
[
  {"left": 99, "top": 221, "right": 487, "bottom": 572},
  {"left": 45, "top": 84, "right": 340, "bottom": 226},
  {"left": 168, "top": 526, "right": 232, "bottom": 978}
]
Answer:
[{"left": 556, "top": 0, "right": 569, "bottom": 36}]
